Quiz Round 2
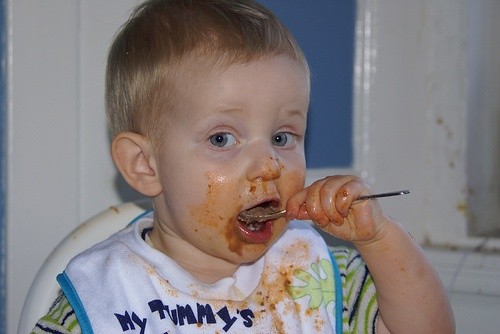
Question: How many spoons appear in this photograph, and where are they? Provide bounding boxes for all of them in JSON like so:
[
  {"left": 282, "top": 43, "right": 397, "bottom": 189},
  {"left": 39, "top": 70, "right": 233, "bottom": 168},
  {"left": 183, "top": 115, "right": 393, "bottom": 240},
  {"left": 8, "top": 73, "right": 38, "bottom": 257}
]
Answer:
[{"left": 240, "top": 189, "right": 410, "bottom": 219}]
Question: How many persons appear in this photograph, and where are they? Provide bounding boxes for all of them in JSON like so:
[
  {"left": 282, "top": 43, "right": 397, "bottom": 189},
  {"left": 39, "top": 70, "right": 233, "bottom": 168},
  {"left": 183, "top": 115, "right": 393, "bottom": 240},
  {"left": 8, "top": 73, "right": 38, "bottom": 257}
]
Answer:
[{"left": 29, "top": 0, "right": 456, "bottom": 334}]
